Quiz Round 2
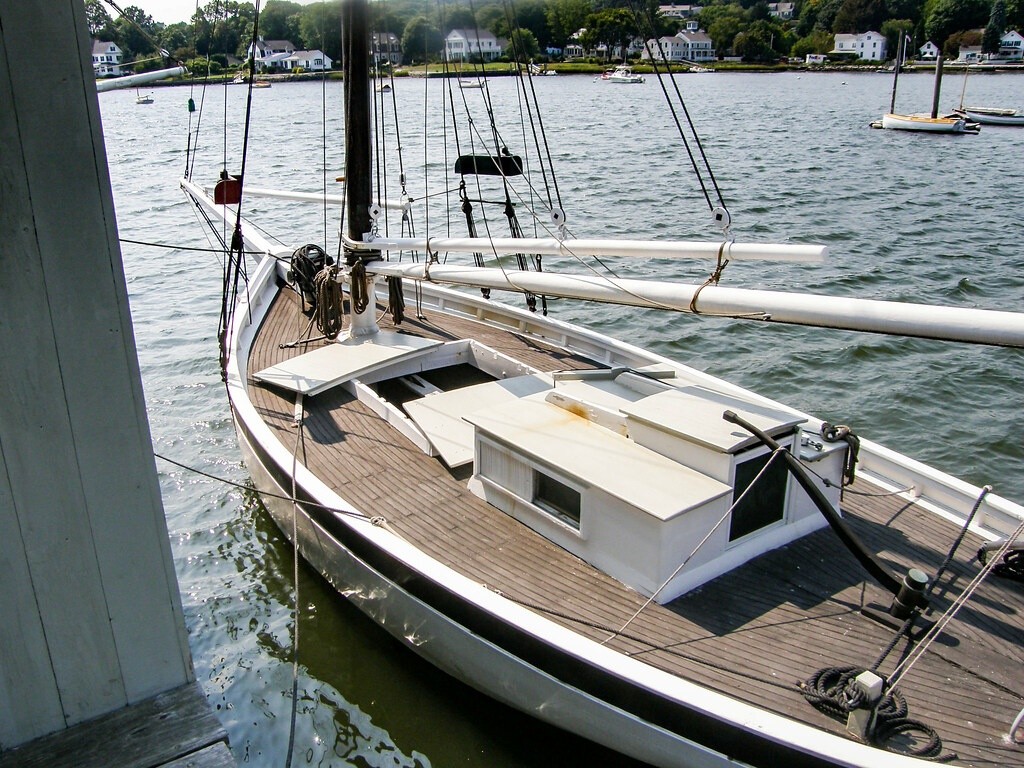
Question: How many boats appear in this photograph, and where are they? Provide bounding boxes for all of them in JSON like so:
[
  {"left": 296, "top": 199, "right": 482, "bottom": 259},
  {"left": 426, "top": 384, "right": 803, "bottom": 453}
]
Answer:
[{"left": 225, "top": 60, "right": 650, "bottom": 92}]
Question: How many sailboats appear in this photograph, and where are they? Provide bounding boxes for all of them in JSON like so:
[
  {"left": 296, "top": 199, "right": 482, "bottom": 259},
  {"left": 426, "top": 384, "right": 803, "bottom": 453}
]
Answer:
[{"left": 175, "top": 4, "right": 1022, "bottom": 768}]
